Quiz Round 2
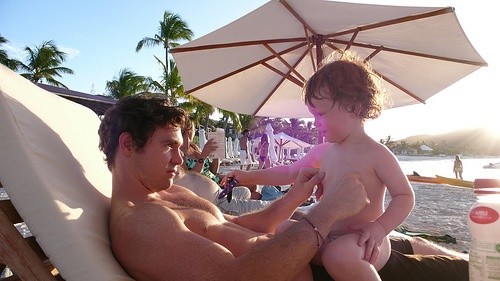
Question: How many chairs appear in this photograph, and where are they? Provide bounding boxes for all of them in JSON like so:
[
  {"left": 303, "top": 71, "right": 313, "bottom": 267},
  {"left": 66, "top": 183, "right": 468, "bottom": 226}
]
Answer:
[{"left": 0, "top": 63, "right": 409, "bottom": 280}]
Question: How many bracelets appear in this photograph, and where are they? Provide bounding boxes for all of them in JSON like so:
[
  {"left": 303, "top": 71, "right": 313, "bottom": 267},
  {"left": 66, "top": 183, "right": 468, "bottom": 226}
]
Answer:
[{"left": 302, "top": 218, "right": 325, "bottom": 248}]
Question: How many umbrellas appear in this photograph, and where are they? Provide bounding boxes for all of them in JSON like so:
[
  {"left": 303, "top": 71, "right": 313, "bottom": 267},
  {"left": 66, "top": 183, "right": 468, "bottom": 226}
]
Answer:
[
  {"left": 253, "top": 131, "right": 311, "bottom": 160},
  {"left": 168, "top": 0, "right": 488, "bottom": 144}
]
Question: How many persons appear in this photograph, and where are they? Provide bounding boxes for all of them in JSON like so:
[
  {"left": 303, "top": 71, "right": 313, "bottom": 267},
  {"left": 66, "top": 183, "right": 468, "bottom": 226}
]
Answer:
[
  {"left": 219, "top": 49, "right": 416, "bottom": 281},
  {"left": 180, "top": 119, "right": 263, "bottom": 200},
  {"left": 96, "top": 91, "right": 470, "bottom": 281},
  {"left": 453, "top": 155, "right": 464, "bottom": 180},
  {"left": 171, "top": 119, "right": 318, "bottom": 216},
  {"left": 239, "top": 128, "right": 252, "bottom": 171},
  {"left": 256, "top": 134, "right": 270, "bottom": 169}
]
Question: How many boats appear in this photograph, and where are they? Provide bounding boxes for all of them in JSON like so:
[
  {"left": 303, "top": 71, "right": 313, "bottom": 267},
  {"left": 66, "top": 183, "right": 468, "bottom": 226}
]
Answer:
[
  {"left": 483, "top": 161, "right": 500, "bottom": 169},
  {"left": 406, "top": 171, "right": 474, "bottom": 188}
]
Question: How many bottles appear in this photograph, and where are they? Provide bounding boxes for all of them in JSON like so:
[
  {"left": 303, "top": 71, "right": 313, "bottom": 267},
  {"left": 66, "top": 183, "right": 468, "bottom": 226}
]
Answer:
[{"left": 469, "top": 178, "right": 500, "bottom": 281}]
